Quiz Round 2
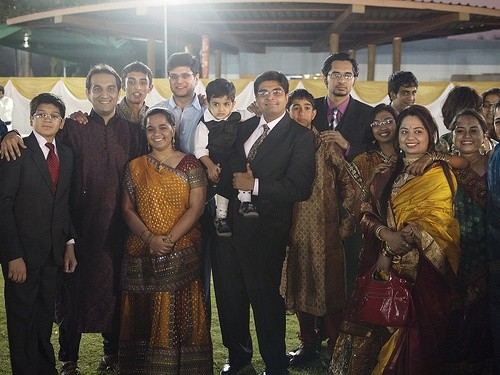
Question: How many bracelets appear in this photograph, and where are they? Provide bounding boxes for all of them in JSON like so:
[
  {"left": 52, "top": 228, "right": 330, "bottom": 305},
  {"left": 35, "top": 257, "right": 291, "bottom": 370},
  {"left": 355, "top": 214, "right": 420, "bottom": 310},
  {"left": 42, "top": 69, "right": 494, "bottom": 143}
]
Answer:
[
  {"left": 385, "top": 244, "right": 396, "bottom": 255},
  {"left": 141, "top": 230, "right": 149, "bottom": 239},
  {"left": 440, "top": 151, "right": 452, "bottom": 162},
  {"left": 434, "top": 150, "right": 441, "bottom": 160},
  {"left": 361, "top": 188, "right": 369, "bottom": 198},
  {"left": 377, "top": 227, "right": 391, "bottom": 242},
  {"left": 381, "top": 248, "right": 391, "bottom": 258},
  {"left": 163, "top": 238, "right": 172, "bottom": 244},
  {"left": 429, "top": 150, "right": 436, "bottom": 161},
  {"left": 424, "top": 152, "right": 432, "bottom": 164},
  {"left": 364, "top": 185, "right": 369, "bottom": 193},
  {"left": 376, "top": 225, "right": 386, "bottom": 236},
  {"left": 359, "top": 194, "right": 367, "bottom": 202},
  {"left": 143, "top": 233, "right": 156, "bottom": 247}
]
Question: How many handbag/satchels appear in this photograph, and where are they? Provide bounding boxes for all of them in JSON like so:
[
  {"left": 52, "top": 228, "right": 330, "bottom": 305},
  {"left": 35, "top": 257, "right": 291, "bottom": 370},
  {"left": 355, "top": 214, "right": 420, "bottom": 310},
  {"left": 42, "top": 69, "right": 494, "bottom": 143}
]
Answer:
[{"left": 345, "top": 264, "right": 418, "bottom": 327}]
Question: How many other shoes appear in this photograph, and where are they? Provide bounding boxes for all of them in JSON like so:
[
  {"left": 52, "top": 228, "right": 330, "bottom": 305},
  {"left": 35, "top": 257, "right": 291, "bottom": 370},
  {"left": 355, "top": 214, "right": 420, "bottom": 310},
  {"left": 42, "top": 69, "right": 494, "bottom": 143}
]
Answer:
[
  {"left": 239, "top": 202, "right": 258, "bottom": 218},
  {"left": 287, "top": 347, "right": 320, "bottom": 364},
  {"left": 60, "top": 362, "right": 82, "bottom": 375},
  {"left": 214, "top": 218, "right": 232, "bottom": 236}
]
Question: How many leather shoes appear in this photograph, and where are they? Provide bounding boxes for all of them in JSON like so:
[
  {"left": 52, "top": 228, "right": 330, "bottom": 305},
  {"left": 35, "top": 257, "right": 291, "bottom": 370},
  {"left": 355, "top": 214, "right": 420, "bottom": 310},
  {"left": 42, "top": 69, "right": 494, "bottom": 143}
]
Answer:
[
  {"left": 220, "top": 358, "right": 251, "bottom": 375},
  {"left": 260, "top": 366, "right": 289, "bottom": 375}
]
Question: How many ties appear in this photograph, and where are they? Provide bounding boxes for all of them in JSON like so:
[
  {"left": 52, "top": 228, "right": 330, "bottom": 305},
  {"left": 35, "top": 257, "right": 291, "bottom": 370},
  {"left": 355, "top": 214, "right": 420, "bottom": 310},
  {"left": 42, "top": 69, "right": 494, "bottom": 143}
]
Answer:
[
  {"left": 246, "top": 124, "right": 270, "bottom": 168},
  {"left": 45, "top": 143, "right": 60, "bottom": 192},
  {"left": 328, "top": 108, "right": 339, "bottom": 132}
]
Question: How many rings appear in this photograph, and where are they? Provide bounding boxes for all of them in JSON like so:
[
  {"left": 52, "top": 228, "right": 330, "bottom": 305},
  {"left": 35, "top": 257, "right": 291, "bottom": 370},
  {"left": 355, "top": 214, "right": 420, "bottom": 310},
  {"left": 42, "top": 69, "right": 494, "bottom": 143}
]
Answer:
[
  {"left": 71, "top": 114, "right": 74, "bottom": 116},
  {"left": 403, "top": 241, "right": 408, "bottom": 247},
  {"left": 380, "top": 269, "right": 383, "bottom": 272}
]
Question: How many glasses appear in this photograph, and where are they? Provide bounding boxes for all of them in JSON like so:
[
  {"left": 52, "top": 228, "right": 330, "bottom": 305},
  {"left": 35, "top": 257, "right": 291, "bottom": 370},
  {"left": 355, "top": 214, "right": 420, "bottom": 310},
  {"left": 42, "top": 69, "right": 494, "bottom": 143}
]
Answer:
[
  {"left": 328, "top": 72, "right": 354, "bottom": 80},
  {"left": 370, "top": 119, "right": 396, "bottom": 128},
  {"left": 33, "top": 112, "right": 62, "bottom": 121},
  {"left": 257, "top": 90, "right": 286, "bottom": 97},
  {"left": 167, "top": 74, "right": 194, "bottom": 81}
]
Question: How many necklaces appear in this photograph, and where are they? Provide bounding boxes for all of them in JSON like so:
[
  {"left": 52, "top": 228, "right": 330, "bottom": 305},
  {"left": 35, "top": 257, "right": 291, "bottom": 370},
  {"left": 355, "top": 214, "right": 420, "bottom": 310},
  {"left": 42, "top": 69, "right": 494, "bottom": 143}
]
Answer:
[{"left": 151, "top": 153, "right": 174, "bottom": 172}]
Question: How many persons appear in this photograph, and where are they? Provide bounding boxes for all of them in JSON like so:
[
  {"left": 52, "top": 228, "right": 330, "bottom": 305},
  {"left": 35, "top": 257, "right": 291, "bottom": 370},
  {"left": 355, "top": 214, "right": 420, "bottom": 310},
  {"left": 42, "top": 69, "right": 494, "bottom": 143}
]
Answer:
[
  {"left": 193, "top": 52, "right": 499, "bottom": 375},
  {"left": 0, "top": 51, "right": 216, "bottom": 375}
]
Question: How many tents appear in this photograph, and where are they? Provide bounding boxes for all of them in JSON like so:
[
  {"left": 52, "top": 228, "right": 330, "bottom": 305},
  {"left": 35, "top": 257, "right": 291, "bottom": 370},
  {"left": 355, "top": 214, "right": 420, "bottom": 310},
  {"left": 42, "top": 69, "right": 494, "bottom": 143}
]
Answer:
[{"left": 3, "top": 79, "right": 456, "bottom": 140}]
